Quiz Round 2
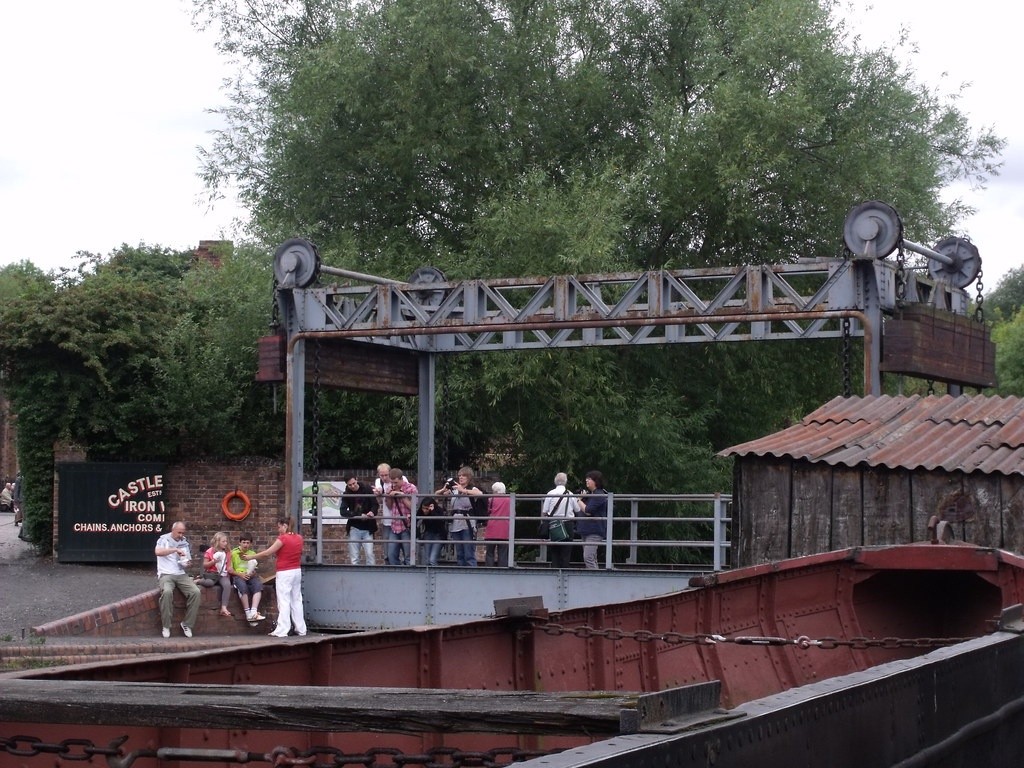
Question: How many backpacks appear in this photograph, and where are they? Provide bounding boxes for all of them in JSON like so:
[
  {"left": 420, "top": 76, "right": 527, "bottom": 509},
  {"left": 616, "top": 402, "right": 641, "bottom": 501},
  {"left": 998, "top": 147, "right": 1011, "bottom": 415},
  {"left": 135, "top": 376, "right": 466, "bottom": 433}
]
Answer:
[{"left": 466, "top": 483, "right": 488, "bottom": 523}]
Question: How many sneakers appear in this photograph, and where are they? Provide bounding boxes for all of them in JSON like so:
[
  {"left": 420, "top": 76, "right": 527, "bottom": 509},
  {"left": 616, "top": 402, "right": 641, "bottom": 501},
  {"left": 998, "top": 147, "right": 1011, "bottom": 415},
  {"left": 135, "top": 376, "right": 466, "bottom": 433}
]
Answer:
[
  {"left": 181, "top": 621, "right": 192, "bottom": 638},
  {"left": 162, "top": 626, "right": 170, "bottom": 638}
]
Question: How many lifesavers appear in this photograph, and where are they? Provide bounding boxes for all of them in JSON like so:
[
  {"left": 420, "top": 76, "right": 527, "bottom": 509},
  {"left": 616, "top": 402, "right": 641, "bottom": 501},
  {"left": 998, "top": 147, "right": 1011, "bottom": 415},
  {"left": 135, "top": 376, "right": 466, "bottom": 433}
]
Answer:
[{"left": 223, "top": 491, "right": 250, "bottom": 519}]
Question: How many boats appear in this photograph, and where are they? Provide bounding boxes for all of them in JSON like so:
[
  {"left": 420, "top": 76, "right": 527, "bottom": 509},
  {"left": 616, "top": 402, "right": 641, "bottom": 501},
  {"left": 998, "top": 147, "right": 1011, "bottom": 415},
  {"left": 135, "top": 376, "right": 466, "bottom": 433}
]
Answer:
[{"left": 1, "top": 521, "right": 1024, "bottom": 767}]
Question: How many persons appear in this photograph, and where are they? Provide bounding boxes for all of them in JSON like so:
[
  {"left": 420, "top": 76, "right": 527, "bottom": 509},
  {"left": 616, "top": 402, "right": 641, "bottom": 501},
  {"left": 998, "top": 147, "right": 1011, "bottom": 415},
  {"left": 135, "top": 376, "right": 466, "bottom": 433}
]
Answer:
[
  {"left": 337, "top": 471, "right": 381, "bottom": 567},
  {"left": 10, "top": 483, "right": 16, "bottom": 500},
  {"left": 576, "top": 470, "right": 616, "bottom": 569},
  {"left": 240, "top": 515, "right": 310, "bottom": 638},
  {"left": 0, "top": 482, "right": 15, "bottom": 509},
  {"left": 385, "top": 468, "right": 420, "bottom": 566},
  {"left": 12, "top": 471, "right": 24, "bottom": 526},
  {"left": 226, "top": 532, "right": 268, "bottom": 628},
  {"left": 151, "top": 519, "right": 202, "bottom": 639},
  {"left": 190, "top": 530, "right": 237, "bottom": 618},
  {"left": 435, "top": 464, "right": 486, "bottom": 564},
  {"left": 480, "top": 482, "right": 517, "bottom": 568},
  {"left": 405, "top": 496, "right": 450, "bottom": 563},
  {"left": 369, "top": 463, "right": 411, "bottom": 566},
  {"left": 540, "top": 473, "right": 585, "bottom": 571}
]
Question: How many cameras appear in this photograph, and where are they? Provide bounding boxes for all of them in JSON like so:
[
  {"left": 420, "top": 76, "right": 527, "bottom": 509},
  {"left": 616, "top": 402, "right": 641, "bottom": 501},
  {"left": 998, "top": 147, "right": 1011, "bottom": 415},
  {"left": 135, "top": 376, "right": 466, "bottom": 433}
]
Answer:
[
  {"left": 575, "top": 488, "right": 591, "bottom": 494},
  {"left": 446, "top": 477, "right": 457, "bottom": 491}
]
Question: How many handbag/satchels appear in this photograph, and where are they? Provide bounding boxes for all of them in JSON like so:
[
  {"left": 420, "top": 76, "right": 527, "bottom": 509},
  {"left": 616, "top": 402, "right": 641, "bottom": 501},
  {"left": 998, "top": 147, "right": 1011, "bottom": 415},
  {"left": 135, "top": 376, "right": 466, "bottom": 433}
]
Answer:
[
  {"left": 548, "top": 519, "right": 569, "bottom": 542},
  {"left": 536, "top": 518, "right": 550, "bottom": 539},
  {"left": 203, "top": 571, "right": 220, "bottom": 580},
  {"left": 477, "top": 518, "right": 486, "bottom": 528}
]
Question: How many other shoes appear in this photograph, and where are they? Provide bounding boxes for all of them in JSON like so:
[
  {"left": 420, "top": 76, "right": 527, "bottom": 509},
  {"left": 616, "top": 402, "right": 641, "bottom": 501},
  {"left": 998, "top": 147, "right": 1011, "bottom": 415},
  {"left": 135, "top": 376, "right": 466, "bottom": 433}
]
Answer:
[
  {"left": 247, "top": 614, "right": 266, "bottom": 627},
  {"left": 269, "top": 632, "right": 288, "bottom": 637},
  {"left": 219, "top": 611, "right": 231, "bottom": 616},
  {"left": 293, "top": 629, "right": 306, "bottom": 636}
]
